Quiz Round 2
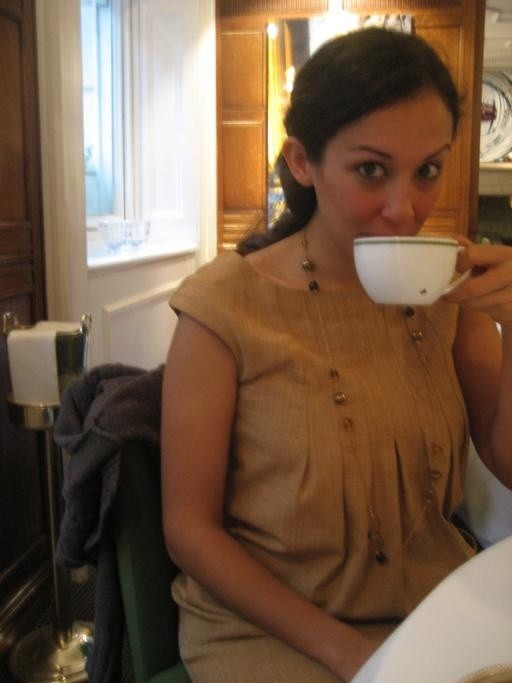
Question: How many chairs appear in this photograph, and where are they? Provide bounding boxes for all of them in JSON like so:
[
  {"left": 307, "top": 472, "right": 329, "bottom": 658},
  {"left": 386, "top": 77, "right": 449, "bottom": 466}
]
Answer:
[{"left": 116, "top": 443, "right": 233, "bottom": 683}]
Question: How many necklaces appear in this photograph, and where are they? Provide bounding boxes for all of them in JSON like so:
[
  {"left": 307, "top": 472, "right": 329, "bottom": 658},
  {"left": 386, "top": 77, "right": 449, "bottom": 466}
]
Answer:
[{"left": 299, "top": 225, "right": 454, "bottom": 568}]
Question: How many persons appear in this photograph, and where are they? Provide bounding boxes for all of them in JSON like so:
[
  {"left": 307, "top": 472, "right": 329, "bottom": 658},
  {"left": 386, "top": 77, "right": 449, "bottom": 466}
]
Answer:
[{"left": 162, "top": 27, "right": 511, "bottom": 683}]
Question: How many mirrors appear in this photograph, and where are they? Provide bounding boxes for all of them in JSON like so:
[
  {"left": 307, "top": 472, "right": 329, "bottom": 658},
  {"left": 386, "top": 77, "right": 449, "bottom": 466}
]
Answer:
[{"left": 266, "top": 13, "right": 412, "bottom": 235}]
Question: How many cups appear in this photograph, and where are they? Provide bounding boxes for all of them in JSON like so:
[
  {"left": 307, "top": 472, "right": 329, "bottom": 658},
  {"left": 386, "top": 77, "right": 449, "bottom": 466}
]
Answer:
[{"left": 351, "top": 233, "right": 476, "bottom": 308}]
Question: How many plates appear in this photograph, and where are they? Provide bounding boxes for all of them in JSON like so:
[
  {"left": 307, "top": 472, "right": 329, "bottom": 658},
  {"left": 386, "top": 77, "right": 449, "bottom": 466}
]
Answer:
[{"left": 480, "top": 64, "right": 511, "bottom": 163}]
